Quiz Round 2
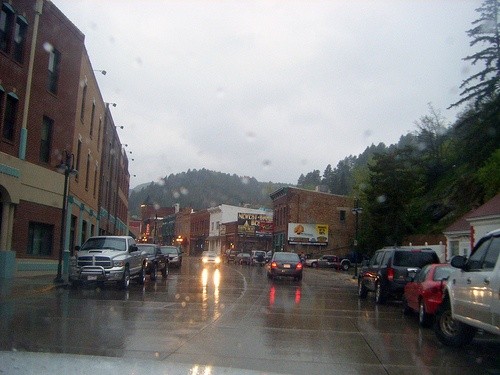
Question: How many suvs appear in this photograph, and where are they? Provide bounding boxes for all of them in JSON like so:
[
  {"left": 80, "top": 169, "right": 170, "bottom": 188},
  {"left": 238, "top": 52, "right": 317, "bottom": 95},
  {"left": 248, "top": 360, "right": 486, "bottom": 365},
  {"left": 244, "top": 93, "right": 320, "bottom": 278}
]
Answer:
[
  {"left": 358, "top": 245, "right": 442, "bottom": 305},
  {"left": 434, "top": 229, "right": 500, "bottom": 349},
  {"left": 74, "top": 235, "right": 152, "bottom": 289},
  {"left": 137, "top": 243, "right": 169, "bottom": 281}
]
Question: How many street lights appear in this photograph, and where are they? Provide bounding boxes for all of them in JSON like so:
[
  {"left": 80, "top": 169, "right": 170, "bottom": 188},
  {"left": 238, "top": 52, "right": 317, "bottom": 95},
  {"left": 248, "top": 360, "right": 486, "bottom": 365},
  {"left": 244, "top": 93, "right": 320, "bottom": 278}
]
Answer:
[
  {"left": 52, "top": 150, "right": 79, "bottom": 285},
  {"left": 352, "top": 198, "right": 364, "bottom": 280},
  {"left": 153, "top": 203, "right": 161, "bottom": 245}
]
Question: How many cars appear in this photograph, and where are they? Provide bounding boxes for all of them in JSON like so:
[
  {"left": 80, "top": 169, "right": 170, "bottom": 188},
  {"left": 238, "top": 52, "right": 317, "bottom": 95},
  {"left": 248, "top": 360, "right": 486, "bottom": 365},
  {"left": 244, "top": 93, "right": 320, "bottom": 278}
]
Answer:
[
  {"left": 201, "top": 251, "right": 221, "bottom": 265},
  {"left": 159, "top": 245, "right": 184, "bottom": 269},
  {"left": 399, "top": 263, "right": 460, "bottom": 327},
  {"left": 305, "top": 255, "right": 351, "bottom": 271},
  {"left": 221, "top": 249, "right": 273, "bottom": 267},
  {"left": 266, "top": 252, "right": 304, "bottom": 280}
]
